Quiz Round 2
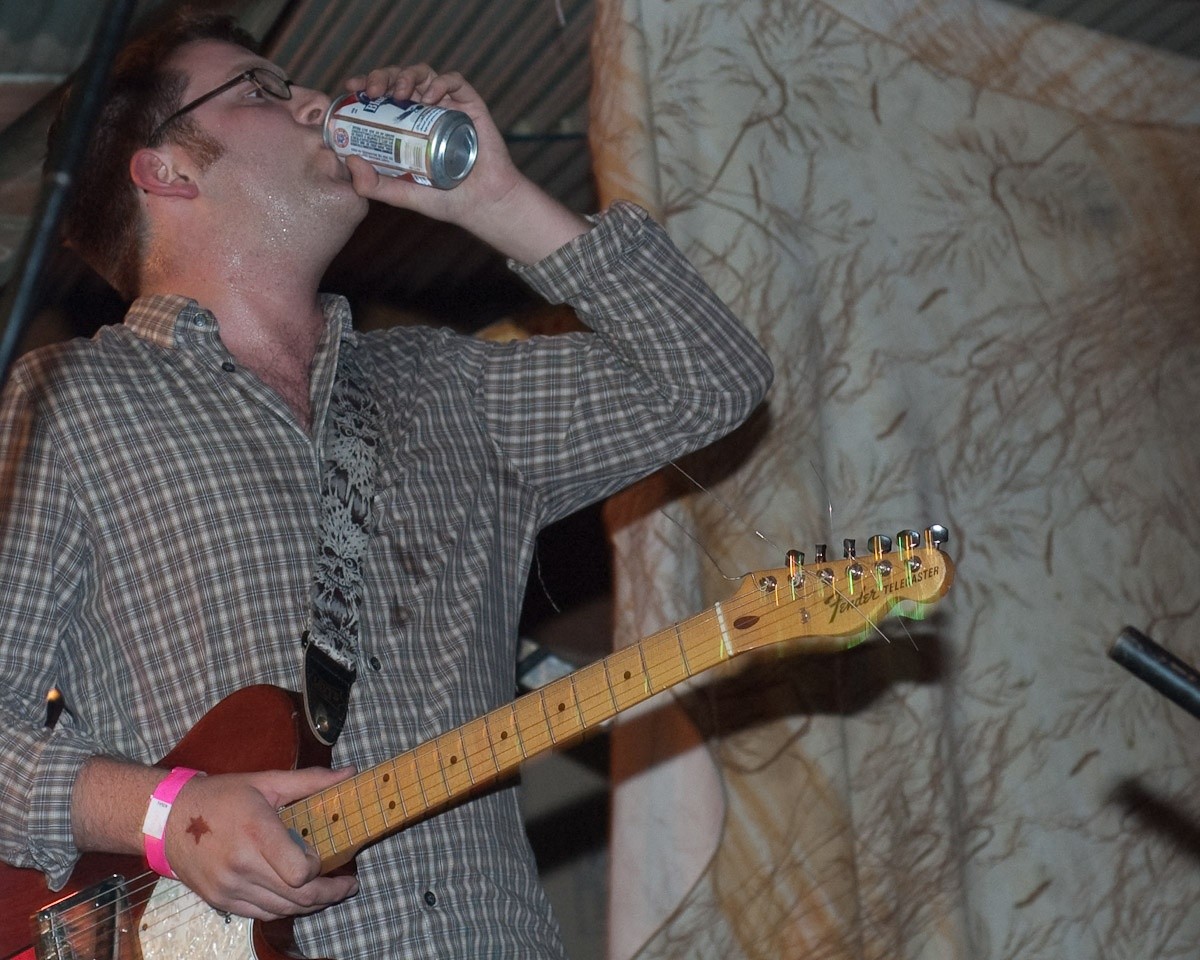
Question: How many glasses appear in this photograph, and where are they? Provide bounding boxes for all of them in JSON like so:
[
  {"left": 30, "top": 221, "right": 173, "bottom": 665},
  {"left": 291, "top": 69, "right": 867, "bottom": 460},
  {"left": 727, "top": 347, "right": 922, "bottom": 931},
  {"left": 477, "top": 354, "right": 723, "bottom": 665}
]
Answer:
[{"left": 141, "top": 67, "right": 294, "bottom": 194}]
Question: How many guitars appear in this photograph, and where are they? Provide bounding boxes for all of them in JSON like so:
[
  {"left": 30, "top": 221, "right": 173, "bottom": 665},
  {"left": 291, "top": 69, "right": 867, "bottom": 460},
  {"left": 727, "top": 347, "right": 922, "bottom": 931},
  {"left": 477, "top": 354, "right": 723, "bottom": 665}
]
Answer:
[{"left": 0, "top": 524, "right": 956, "bottom": 960}]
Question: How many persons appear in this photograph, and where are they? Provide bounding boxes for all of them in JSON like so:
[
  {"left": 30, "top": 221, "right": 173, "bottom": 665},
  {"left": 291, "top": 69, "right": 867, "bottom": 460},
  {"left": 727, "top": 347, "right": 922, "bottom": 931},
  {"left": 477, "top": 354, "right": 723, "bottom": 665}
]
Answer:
[{"left": 0, "top": 0, "right": 775, "bottom": 960}]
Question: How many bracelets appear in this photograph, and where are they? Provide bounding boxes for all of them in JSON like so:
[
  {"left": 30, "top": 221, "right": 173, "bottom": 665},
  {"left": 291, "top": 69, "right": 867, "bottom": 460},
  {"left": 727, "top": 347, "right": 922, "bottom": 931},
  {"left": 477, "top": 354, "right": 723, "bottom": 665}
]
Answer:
[{"left": 142, "top": 766, "right": 204, "bottom": 882}]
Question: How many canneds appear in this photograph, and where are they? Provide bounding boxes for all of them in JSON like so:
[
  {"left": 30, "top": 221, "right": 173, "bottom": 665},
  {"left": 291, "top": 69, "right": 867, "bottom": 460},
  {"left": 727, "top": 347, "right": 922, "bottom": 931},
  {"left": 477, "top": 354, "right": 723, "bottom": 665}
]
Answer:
[{"left": 323, "top": 92, "right": 478, "bottom": 191}]
